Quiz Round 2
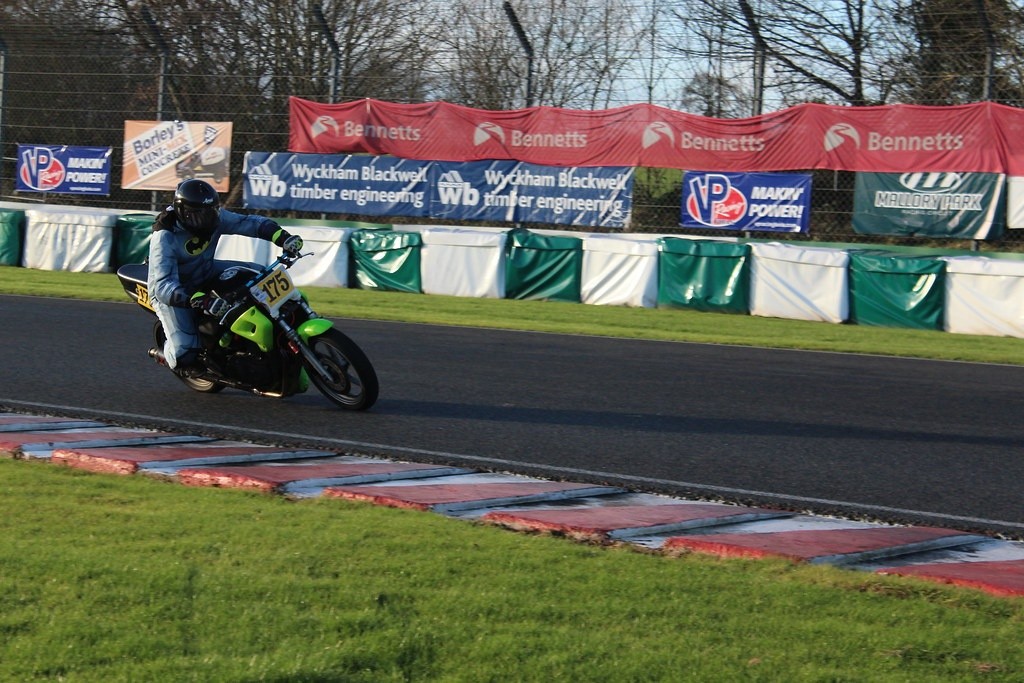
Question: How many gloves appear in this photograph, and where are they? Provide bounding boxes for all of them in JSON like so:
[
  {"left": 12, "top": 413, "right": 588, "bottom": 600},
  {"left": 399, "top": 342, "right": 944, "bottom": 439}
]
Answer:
[
  {"left": 208, "top": 299, "right": 227, "bottom": 316},
  {"left": 284, "top": 235, "right": 303, "bottom": 259}
]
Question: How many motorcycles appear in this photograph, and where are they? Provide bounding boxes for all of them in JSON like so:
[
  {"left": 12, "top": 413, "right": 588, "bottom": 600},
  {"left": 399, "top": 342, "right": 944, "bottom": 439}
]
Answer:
[{"left": 117, "top": 249, "right": 380, "bottom": 411}]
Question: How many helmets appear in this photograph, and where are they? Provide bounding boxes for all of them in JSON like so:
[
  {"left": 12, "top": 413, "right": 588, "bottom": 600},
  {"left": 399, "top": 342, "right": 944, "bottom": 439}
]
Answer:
[{"left": 176, "top": 180, "right": 220, "bottom": 233}]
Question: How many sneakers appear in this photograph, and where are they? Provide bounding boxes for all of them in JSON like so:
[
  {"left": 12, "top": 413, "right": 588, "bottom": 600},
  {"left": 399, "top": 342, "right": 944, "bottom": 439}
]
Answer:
[{"left": 178, "top": 358, "right": 207, "bottom": 378}]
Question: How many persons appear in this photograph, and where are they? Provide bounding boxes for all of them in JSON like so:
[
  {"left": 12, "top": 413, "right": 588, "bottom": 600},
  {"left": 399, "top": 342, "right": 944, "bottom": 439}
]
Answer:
[{"left": 148, "top": 179, "right": 302, "bottom": 372}]
